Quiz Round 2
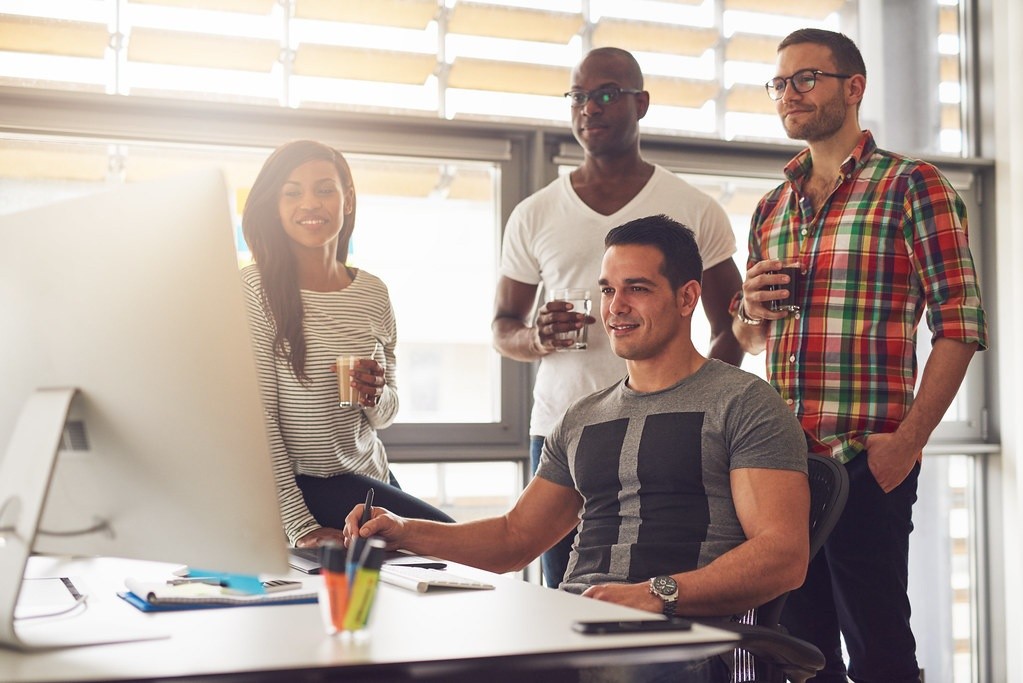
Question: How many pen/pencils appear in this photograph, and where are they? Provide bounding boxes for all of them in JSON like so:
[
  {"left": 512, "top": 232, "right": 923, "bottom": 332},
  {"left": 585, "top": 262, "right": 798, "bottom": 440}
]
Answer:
[
  {"left": 165, "top": 577, "right": 226, "bottom": 584},
  {"left": 390, "top": 561, "right": 448, "bottom": 569}
]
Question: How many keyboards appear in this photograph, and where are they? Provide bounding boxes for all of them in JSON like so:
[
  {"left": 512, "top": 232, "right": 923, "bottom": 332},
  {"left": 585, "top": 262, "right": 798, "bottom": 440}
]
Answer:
[{"left": 375, "top": 564, "right": 494, "bottom": 594}]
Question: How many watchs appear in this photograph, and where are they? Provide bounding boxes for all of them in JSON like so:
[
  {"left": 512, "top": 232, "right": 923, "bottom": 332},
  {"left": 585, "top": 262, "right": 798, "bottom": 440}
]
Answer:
[
  {"left": 648, "top": 575, "right": 679, "bottom": 618},
  {"left": 737, "top": 298, "right": 763, "bottom": 326}
]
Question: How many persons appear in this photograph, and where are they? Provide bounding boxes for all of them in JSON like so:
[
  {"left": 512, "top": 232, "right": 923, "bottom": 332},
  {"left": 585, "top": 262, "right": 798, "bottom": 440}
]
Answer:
[
  {"left": 239, "top": 139, "right": 459, "bottom": 549},
  {"left": 343, "top": 215, "right": 812, "bottom": 629},
  {"left": 731, "top": 29, "right": 987, "bottom": 683},
  {"left": 492, "top": 47, "right": 745, "bottom": 588}
]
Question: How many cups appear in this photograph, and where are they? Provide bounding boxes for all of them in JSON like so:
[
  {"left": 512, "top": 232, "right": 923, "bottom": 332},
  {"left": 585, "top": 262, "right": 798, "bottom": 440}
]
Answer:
[
  {"left": 316, "top": 576, "right": 381, "bottom": 634},
  {"left": 552, "top": 288, "right": 593, "bottom": 350},
  {"left": 336, "top": 355, "right": 370, "bottom": 407},
  {"left": 767, "top": 258, "right": 802, "bottom": 312}
]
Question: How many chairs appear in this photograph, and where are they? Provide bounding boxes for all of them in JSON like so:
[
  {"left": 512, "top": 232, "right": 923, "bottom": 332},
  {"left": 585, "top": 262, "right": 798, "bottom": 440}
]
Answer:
[{"left": 706, "top": 454, "right": 849, "bottom": 683}]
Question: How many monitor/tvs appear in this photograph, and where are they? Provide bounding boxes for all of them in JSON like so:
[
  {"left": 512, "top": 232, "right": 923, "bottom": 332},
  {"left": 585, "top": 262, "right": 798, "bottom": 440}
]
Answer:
[{"left": 1, "top": 167, "right": 291, "bottom": 653}]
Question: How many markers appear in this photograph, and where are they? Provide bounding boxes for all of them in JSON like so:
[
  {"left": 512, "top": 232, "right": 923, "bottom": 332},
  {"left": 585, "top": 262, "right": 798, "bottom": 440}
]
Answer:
[
  {"left": 316, "top": 536, "right": 387, "bottom": 633},
  {"left": 359, "top": 488, "right": 375, "bottom": 533}
]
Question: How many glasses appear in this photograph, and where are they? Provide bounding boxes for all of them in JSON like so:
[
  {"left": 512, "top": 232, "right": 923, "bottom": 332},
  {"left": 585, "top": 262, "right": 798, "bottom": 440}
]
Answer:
[
  {"left": 766, "top": 69, "right": 852, "bottom": 101},
  {"left": 564, "top": 86, "right": 642, "bottom": 107}
]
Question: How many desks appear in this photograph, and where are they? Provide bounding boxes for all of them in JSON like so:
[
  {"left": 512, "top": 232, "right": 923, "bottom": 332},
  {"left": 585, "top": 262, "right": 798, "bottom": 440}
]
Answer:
[{"left": 0, "top": 558, "right": 745, "bottom": 683}]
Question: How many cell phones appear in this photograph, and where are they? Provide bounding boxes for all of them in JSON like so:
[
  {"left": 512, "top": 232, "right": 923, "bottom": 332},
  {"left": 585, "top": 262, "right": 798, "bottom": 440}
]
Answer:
[{"left": 572, "top": 615, "right": 692, "bottom": 632}]
